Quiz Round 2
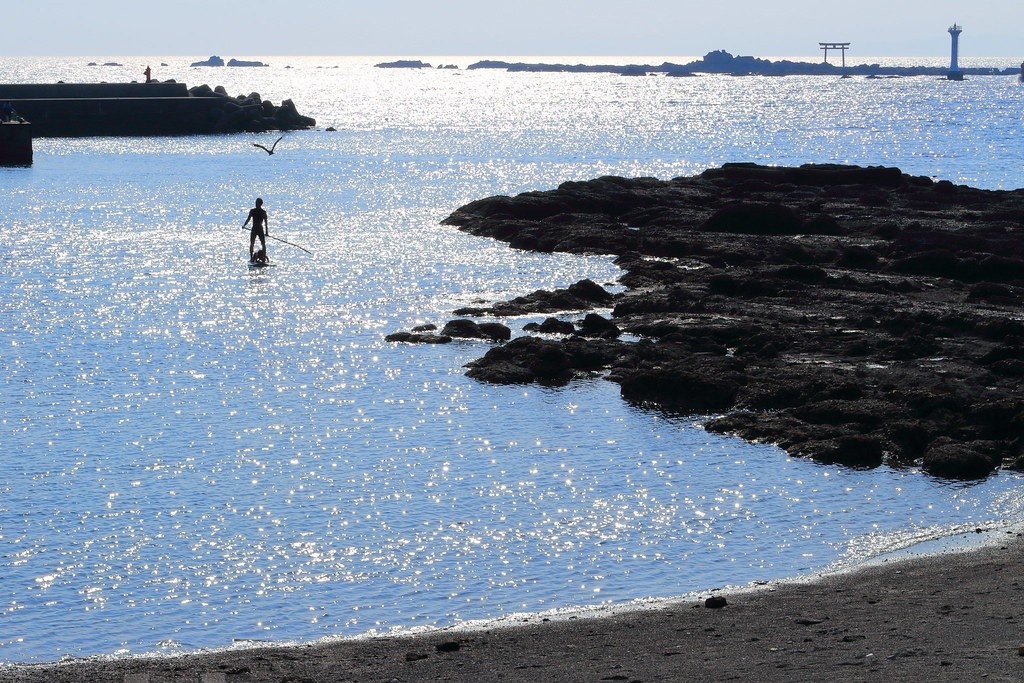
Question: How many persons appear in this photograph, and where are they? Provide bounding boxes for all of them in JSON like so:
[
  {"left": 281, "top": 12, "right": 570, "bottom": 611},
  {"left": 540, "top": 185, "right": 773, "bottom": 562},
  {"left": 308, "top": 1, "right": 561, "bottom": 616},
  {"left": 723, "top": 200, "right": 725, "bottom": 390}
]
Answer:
[{"left": 242, "top": 198, "right": 269, "bottom": 262}]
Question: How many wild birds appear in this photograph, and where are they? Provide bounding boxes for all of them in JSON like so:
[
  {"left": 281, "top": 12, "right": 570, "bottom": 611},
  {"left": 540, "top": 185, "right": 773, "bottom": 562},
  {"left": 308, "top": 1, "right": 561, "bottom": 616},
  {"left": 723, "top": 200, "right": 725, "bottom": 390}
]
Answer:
[{"left": 253, "top": 135, "right": 282, "bottom": 156}]
